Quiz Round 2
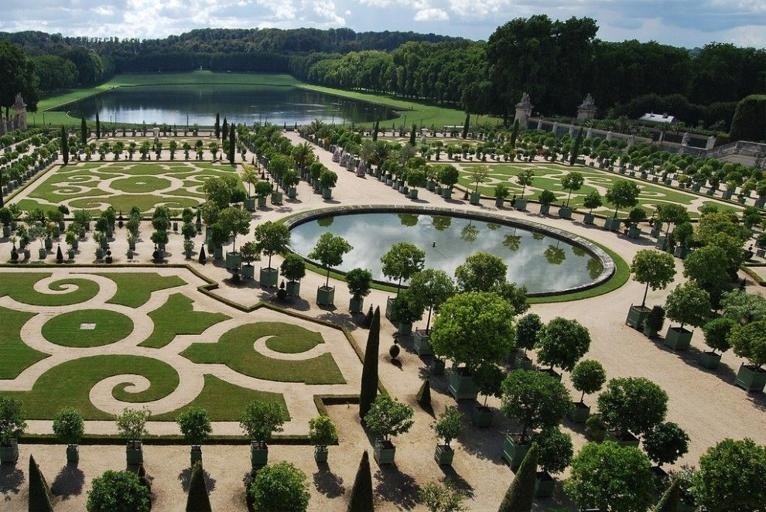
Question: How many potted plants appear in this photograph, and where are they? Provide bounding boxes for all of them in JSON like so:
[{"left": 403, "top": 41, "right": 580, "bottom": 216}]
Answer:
[
  {"left": 462, "top": 112, "right": 667, "bottom": 167},
  {"left": 240, "top": 220, "right": 352, "bottom": 307},
  {"left": 558, "top": 172, "right": 586, "bottom": 217},
  {"left": 607, "top": 147, "right": 762, "bottom": 194},
  {"left": 250, "top": 461, "right": 311, "bottom": 512},
  {"left": 86, "top": 468, "right": 152, "bottom": 512},
  {"left": 115, "top": 404, "right": 151, "bottom": 466},
  {"left": 309, "top": 416, "right": 339, "bottom": 461},
  {"left": 91, "top": 113, "right": 249, "bottom": 162},
  {"left": 603, "top": 178, "right": 641, "bottom": 230},
  {"left": 515, "top": 170, "right": 534, "bottom": 213},
  {"left": 494, "top": 183, "right": 509, "bottom": 210},
  {"left": 469, "top": 166, "right": 489, "bottom": 205},
  {"left": 177, "top": 405, "right": 211, "bottom": 470},
  {"left": 625, "top": 205, "right": 762, "bottom": 395},
  {"left": 53, "top": 406, "right": 86, "bottom": 469},
  {"left": 583, "top": 189, "right": 605, "bottom": 226},
  {"left": 348, "top": 241, "right": 765, "bottom": 512},
  {"left": 2, "top": 204, "right": 251, "bottom": 268},
  {"left": 240, "top": 398, "right": 286, "bottom": 469},
  {"left": 0, "top": 117, "right": 90, "bottom": 196},
  {"left": 300, "top": 109, "right": 459, "bottom": 200},
  {"left": 238, "top": 120, "right": 338, "bottom": 205},
  {"left": 538, "top": 188, "right": 557, "bottom": 216},
  {"left": 1, "top": 398, "right": 29, "bottom": 464}
]
